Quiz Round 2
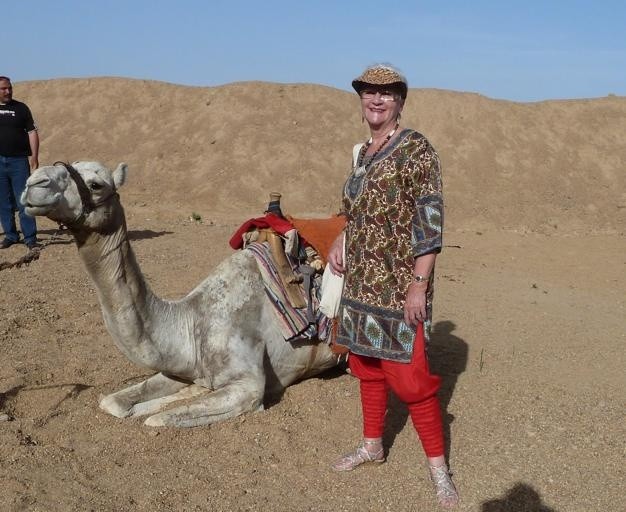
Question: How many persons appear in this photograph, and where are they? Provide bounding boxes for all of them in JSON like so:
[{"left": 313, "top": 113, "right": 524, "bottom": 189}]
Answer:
[
  {"left": 329, "top": 62, "right": 456, "bottom": 502},
  {"left": 1, "top": 76, "right": 39, "bottom": 253}
]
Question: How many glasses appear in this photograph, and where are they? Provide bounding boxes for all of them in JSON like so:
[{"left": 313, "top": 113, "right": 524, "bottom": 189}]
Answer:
[{"left": 357, "top": 87, "right": 402, "bottom": 103}]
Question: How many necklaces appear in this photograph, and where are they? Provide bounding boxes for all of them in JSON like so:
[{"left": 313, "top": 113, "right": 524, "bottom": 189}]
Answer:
[{"left": 354, "top": 124, "right": 398, "bottom": 178}]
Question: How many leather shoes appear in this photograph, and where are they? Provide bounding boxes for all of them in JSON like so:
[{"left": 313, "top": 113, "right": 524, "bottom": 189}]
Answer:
[
  {"left": 0, "top": 238, "right": 13, "bottom": 249},
  {"left": 26, "top": 243, "right": 40, "bottom": 251}
]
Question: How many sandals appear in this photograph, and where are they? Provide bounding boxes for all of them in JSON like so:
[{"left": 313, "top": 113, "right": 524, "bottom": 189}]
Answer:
[
  {"left": 331, "top": 440, "right": 386, "bottom": 472},
  {"left": 426, "top": 461, "right": 460, "bottom": 507}
]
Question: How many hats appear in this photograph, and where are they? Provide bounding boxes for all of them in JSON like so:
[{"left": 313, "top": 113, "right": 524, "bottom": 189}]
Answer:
[{"left": 351, "top": 64, "right": 408, "bottom": 98}]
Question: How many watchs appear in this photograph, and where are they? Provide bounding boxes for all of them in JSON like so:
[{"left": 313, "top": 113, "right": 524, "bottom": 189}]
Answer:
[{"left": 411, "top": 272, "right": 434, "bottom": 284}]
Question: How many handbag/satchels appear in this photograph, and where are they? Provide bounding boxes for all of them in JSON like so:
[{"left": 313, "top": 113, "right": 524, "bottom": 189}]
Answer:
[{"left": 319, "top": 261, "right": 344, "bottom": 320}]
